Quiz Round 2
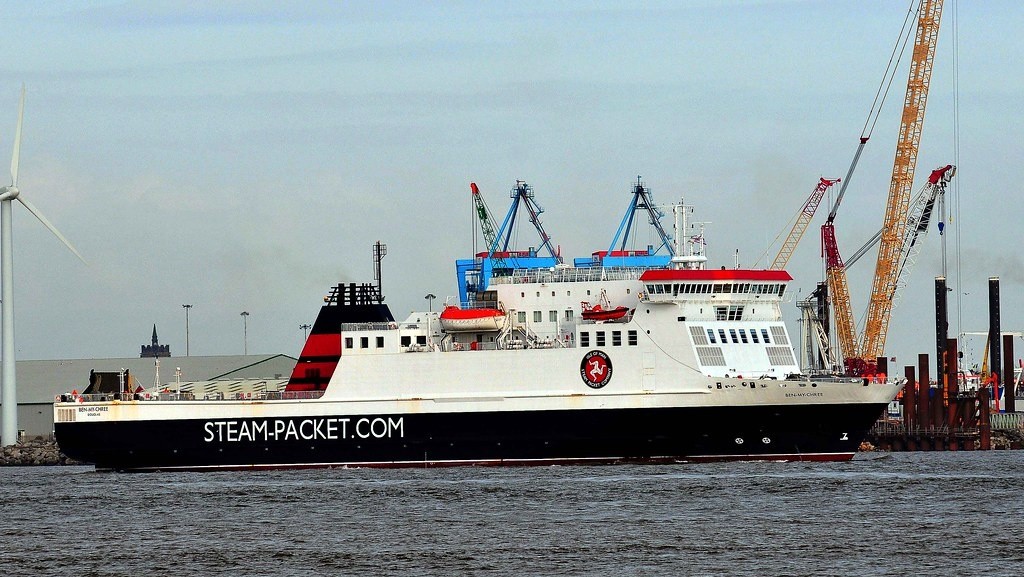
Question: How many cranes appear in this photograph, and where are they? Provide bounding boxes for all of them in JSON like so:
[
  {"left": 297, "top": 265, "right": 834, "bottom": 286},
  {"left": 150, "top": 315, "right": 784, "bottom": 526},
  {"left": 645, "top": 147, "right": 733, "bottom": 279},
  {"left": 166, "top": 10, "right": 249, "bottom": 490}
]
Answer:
[{"left": 774, "top": 0, "right": 960, "bottom": 382}]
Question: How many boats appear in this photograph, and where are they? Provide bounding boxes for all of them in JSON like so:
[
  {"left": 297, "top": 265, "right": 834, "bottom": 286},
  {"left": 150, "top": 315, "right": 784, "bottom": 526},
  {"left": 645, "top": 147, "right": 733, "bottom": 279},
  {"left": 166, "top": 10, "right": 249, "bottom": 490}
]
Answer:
[
  {"left": 52, "top": 173, "right": 908, "bottom": 464},
  {"left": 441, "top": 306, "right": 507, "bottom": 332},
  {"left": 578, "top": 305, "right": 631, "bottom": 320},
  {"left": 987, "top": 367, "right": 1024, "bottom": 411}
]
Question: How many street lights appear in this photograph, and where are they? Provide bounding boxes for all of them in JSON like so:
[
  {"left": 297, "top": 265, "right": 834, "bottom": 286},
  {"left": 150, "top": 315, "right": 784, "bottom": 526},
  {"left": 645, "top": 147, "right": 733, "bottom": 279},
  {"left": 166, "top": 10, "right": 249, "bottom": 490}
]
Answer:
[
  {"left": 241, "top": 312, "right": 250, "bottom": 355},
  {"left": 424, "top": 294, "right": 437, "bottom": 312},
  {"left": 299, "top": 324, "right": 311, "bottom": 341},
  {"left": 182, "top": 304, "right": 193, "bottom": 356}
]
